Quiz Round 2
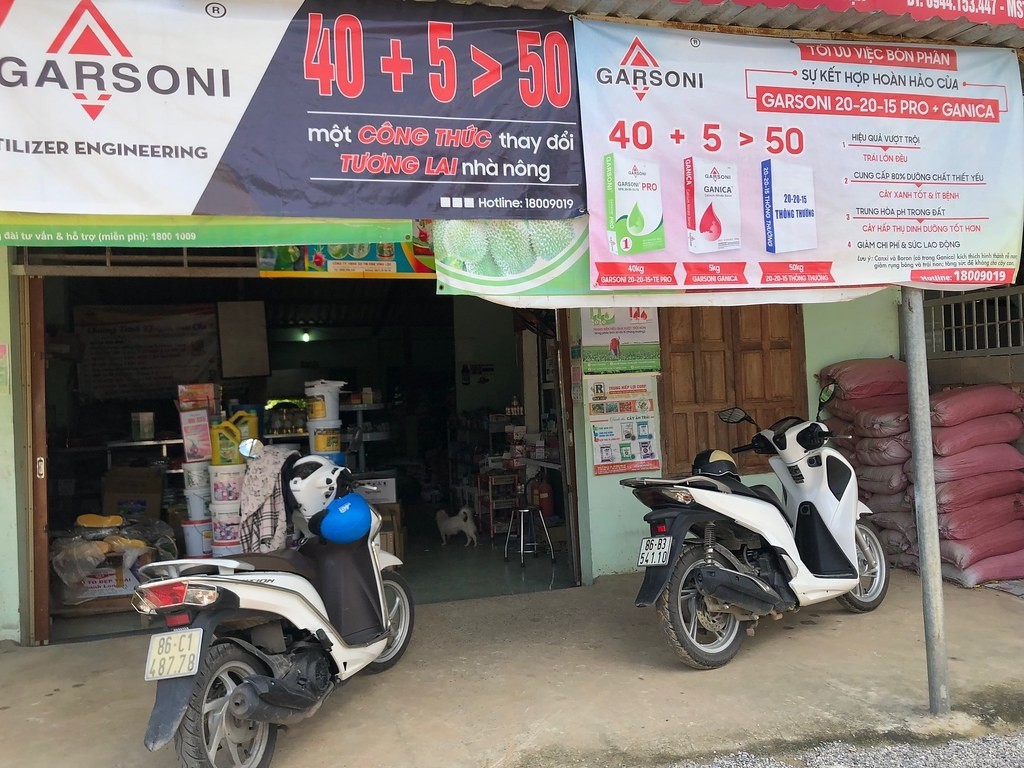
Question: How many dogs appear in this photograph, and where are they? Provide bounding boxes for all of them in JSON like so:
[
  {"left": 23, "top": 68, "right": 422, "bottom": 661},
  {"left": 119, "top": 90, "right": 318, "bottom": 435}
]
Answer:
[{"left": 435, "top": 507, "right": 478, "bottom": 547}]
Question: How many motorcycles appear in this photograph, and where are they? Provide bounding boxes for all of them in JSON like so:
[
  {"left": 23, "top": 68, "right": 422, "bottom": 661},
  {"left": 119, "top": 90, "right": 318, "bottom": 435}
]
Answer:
[
  {"left": 618, "top": 383, "right": 890, "bottom": 672},
  {"left": 130, "top": 427, "right": 415, "bottom": 768}
]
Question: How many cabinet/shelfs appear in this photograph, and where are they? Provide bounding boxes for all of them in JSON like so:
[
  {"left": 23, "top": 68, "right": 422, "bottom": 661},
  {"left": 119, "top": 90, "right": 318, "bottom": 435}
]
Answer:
[
  {"left": 340, "top": 400, "right": 397, "bottom": 472},
  {"left": 475, "top": 472, "right": 521, "bottom": 542}
]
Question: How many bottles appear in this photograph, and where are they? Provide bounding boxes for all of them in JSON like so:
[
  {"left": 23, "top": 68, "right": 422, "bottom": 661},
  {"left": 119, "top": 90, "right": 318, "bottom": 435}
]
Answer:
[
  {"left": 511, "top": 393, "right": 519, "bottom": 407},
  {"left": 210, "top": 420, "right": 242, "bottom": 466},
  {"left": 229, "top": 408, "right": 258, "bottom": 442}
]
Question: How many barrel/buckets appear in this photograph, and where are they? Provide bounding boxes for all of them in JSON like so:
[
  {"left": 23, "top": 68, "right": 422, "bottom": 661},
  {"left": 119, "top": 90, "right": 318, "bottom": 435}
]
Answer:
[
  {"left": 305, "top": 420, "right": 342, "bottom": 454},
  {"left": 183, "top": 488, "right": 211, "bottom": 520},
  {"left": 305, "top": 387, "right": 340, "bottom": 421},
  {"left": 211, "top": 542, "right": 243, "bottom": 558},
  {"left": 208, "top": 464, "right": 247, "bottom": 504},
  {"left": 182, "top": 462, "right": 210, "bottom": 490},
  {"left": 209, "top": 504, "right": 240, "bottom": 541},
  {"left": 180, "top": 519, "right": 213, "bottom": 556}
]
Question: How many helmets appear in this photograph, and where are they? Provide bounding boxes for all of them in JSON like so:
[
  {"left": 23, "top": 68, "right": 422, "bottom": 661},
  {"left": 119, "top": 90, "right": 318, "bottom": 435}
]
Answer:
[
  {"left": 692, "top": 449, "right": 742, "bottom": 483},
  {"left": 308, "top": 492, "right": 371, "bottom": 543}
]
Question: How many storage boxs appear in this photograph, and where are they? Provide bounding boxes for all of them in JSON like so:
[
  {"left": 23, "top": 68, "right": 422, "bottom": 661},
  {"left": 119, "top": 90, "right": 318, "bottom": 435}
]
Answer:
[
  {"left": 479, "top": 425, "right": 544, "bottom": 482},
  {"left": 374, "top": 498, "right": 406, "bottom": 533},
  {"left": 535, "top": 519, "right": 567, "bottom": 542},
  {"left": 380, "top": 530, "right": 396, "bottom": 572},
  {"left": 61, "top": 549, "right": 154, "bottom": 599},
  {"left": 177, "top": 383, "right": 223, "bottom": 415},
  {"left": 101, "top": 467, "right": 168, "bottom": 520},
  {"left": 395, "top": 525, "right": 407, "bottom": 568},
  {"left": 353, "top": 470, "right": 400, "bottom": 505},
  {"left": 172, "top": 395, "right": 213, "bottom": 462}
]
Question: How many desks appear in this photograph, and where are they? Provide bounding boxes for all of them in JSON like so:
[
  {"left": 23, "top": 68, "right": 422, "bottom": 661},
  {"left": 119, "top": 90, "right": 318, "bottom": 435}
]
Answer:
[
  {"left": 519, "top": 457, "right": 560, "bottom": 471},
  {"left": 105, "top": 438, "right": 185, "bottom": 473}
]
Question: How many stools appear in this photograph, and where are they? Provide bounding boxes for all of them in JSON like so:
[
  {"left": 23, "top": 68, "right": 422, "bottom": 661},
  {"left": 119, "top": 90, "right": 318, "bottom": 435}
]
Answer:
[{"left": 504, "top": 504, "right": 556, "bottom": 569}]
